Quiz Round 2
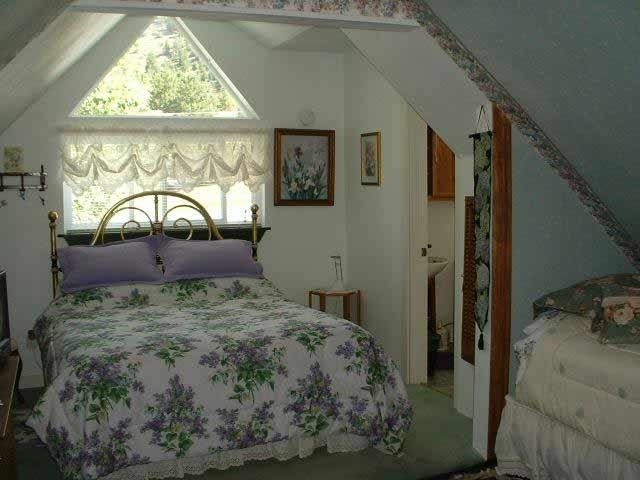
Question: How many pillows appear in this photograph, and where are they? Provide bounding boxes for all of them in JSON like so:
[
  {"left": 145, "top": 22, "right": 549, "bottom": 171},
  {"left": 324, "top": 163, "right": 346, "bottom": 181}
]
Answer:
[
  {"left": 157, "top": 232, "right": 265, "bottom": 283},
  {"left": 55, "top": 234, "right": 164, "bottom": 296}
]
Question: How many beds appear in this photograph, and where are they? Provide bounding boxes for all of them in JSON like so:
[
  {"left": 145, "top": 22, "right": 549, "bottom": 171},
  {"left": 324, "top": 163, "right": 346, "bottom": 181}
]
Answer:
[
  {"left": 495, "top": 272, "right": 639, "bottom": 480},
  {"left": 25, "top": 190, "right": 414, "bottom": 480}
]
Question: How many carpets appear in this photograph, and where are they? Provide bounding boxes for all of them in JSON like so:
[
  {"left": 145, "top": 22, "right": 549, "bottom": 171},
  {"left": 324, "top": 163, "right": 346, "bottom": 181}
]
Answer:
[{"left": 14, "top": 382, "right": 486, "bottom": 480}]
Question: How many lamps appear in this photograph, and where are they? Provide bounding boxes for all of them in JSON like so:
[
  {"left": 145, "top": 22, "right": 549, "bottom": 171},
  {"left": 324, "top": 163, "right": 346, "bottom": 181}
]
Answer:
[{"left": 330, "top": 255, "right": 347, "bottom": 291}]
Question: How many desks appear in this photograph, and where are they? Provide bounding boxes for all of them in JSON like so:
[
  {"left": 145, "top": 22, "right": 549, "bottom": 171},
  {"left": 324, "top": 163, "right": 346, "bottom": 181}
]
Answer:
[{"left": 309, "top": 287, "right": 361, "bottom": 327}]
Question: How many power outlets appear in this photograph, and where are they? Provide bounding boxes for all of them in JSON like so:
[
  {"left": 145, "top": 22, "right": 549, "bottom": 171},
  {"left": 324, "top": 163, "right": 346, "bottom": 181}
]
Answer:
[{"left": 26, "top": 330, "right": 38, "bottom": 350}]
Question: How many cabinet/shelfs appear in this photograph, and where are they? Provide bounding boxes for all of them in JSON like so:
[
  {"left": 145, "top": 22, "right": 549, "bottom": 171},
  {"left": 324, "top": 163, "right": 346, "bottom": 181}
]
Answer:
[
  {"left": 0, "top": 166, "right": 48, "bottom": 191},
  {"left": 428, "top": 125, "right": 455, "bottom": 200},
  {"left": 0, "top": 355, "right": 23, "bottom": 480}
]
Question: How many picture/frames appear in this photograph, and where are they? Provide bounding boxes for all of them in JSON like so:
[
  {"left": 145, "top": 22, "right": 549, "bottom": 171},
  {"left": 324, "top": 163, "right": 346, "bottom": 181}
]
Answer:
[
  {"left": 361, "top": 132, "right": 381, "bottom": 186},
  {"left": 274, "top": 127, "right": 336, "bottom": 207}
]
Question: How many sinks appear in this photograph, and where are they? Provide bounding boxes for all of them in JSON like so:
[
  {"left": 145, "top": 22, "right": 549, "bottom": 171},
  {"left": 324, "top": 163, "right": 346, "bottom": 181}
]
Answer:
[{"left": 427, "top": 257, "right": 448, "bottom": 278}]
